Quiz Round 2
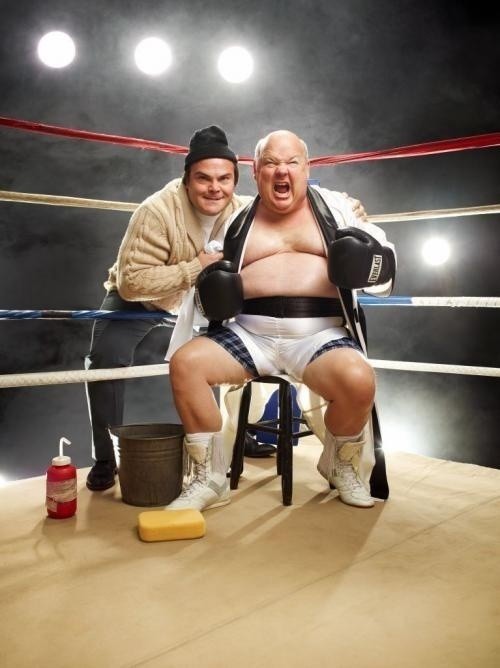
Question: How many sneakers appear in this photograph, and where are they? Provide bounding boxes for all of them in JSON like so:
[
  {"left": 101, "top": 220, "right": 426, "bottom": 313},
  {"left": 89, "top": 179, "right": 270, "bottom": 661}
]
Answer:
[
  {"left": 317, "top": 423, "right": 375, "bottom": 509},
  {"left": 158, "top": 426, "right": 233, "bottom": 515}
]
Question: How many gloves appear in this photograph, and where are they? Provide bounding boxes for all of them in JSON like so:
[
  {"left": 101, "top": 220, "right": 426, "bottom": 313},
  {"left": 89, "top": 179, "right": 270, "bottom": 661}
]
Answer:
[
  {"left": 327, "top": 222, "right": 396, "bottom": 290},
  {"left": 189, "top": 259, "right": 244, "bottom": 321}
]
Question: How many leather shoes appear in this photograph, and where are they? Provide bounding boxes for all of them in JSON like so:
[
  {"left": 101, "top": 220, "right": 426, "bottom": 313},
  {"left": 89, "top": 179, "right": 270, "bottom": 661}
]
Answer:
[
  {"left": 244, "top": 432, "right": 277, "bottom": 458},
  {"left": 85, "top": 457, "right": 120, "bottom": 491}
]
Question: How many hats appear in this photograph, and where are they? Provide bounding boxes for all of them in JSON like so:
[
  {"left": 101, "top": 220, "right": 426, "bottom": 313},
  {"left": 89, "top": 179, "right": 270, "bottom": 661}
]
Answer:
[{"left": 184, "top": 125, "right": 238, "bottom": 168}]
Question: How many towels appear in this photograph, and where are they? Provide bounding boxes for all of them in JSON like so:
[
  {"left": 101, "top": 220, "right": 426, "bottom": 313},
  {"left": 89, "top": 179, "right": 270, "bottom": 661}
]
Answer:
[{"left": 163, "top": 238, "right": 224, "bottom": 364}]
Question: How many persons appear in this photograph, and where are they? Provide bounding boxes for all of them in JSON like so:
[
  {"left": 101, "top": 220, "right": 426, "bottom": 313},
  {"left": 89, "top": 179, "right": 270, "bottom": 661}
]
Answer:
[
  {"left": 162, "top": 127, "right": 399, "bottom": 509},
  {"left": 83, "top": 125, "right": 370, "bottom": 493}
]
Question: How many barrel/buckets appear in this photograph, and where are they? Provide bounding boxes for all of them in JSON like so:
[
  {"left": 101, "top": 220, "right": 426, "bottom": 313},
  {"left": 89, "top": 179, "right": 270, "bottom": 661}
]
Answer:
[{"left": 106, "top": 422, "right": 187, "bottom": 507}]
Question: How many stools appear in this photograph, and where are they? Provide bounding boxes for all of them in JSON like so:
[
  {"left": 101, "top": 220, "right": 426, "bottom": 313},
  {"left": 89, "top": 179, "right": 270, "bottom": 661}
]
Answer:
[{"left": 229, "top": 373, "right": 336, "bottom": 504}]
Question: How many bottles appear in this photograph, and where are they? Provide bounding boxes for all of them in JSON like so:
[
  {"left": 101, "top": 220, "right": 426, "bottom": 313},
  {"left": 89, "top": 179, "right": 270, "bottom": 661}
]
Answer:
[{"left": 45, "top": 456, "right": 77, "bottom": 519}]
